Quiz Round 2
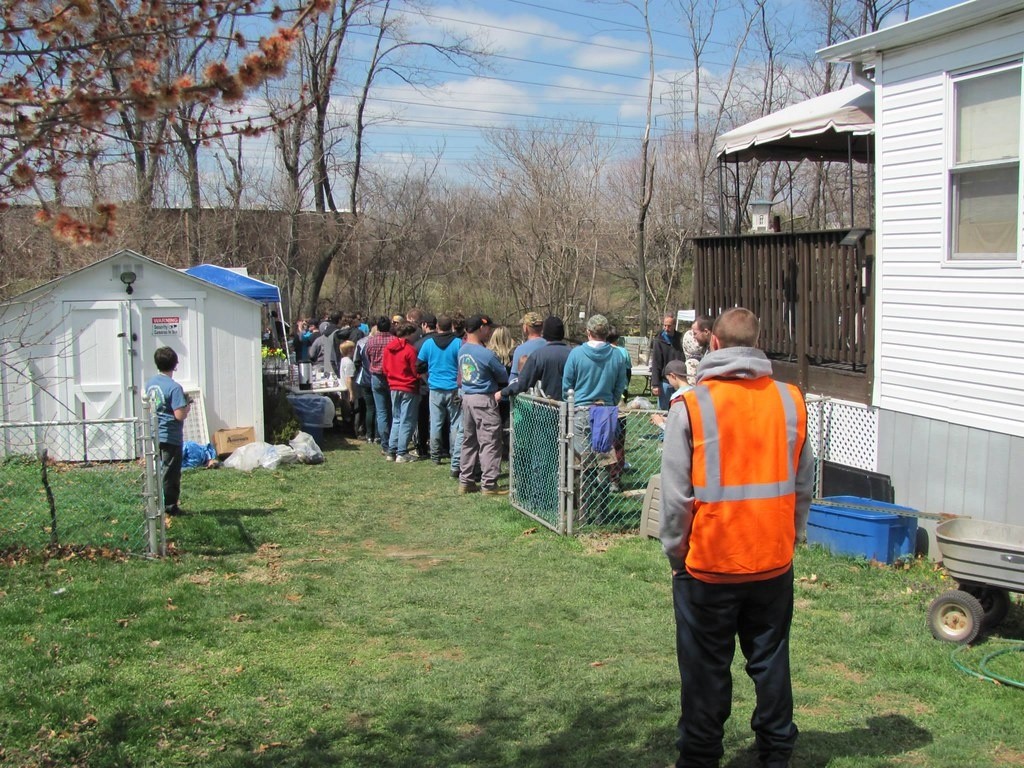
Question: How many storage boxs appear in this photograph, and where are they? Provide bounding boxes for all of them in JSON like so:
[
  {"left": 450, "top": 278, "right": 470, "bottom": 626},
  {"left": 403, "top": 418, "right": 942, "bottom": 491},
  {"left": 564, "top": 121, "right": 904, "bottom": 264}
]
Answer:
[
  {"left": 807, "top": 496, "right": 917, "bottom": 567},
  {"left": 210, "top": 427, "right": 255, "bottom": 458},
  {"left": 287, "top": 396, "right": 327, "bottom": 451}
]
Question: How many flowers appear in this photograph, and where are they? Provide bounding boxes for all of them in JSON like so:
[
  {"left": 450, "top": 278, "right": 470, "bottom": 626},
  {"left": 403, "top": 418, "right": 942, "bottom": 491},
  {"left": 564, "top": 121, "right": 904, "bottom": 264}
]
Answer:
[{"left": 262, "top": 346, "right": 286, "bottom": 359}]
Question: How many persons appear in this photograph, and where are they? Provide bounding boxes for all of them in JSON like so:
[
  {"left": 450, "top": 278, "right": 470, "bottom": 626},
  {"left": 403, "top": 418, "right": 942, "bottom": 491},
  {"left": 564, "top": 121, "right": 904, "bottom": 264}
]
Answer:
[
  {"left": 651, "top": 312, "right": 714, "bottom": 433},
  {"left": 606, "top": 322, "right": 631, "bottom": 490},
  {"left": 563, "top": 314, "right": 627, "bottom": 525},
  {"left": 141, "top": 346, "right": 192, "bottom": 516},
  {"left": 264, "top": 306, "right": 516, "bottom": 481},
  {"left": 508, "top": 311, "right": 547, "bottom": 394},
  {"left": 656, "top": 308, "right": 816, "bottom": 768},
  {"left": 455, "top": 316, "right": 510, "bottom": 497},
  {"left": 493, "top": 316, "right": 574, "bottom": 403}
]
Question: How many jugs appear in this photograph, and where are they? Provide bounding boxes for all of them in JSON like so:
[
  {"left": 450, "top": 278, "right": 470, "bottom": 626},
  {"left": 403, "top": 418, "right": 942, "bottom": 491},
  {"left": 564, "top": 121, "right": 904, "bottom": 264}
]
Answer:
[{"left": 298, "top": 361, "right": 313, "bottom": 390}]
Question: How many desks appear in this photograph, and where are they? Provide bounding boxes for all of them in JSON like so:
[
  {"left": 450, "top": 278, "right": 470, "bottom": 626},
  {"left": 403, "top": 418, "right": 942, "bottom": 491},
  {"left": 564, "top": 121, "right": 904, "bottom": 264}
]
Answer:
[{"left": 278, "top": 380, "right": 347, "bottom": 395}]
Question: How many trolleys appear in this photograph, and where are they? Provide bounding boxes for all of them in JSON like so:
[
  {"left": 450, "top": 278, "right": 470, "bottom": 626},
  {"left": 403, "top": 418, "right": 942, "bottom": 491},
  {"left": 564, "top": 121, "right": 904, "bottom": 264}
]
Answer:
[{"left": 927, "top": 517, "right": 1024, "bottom": 642}]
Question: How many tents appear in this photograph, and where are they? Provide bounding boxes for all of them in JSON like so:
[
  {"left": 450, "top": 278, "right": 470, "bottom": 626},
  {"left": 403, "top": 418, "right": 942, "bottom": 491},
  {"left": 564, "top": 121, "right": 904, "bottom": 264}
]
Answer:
[
  {"left": 183, "top": 262, "right": 291, "bottom": 378},
  {"left": 717, "top": 79, "right": 878, "bottom": 233}
]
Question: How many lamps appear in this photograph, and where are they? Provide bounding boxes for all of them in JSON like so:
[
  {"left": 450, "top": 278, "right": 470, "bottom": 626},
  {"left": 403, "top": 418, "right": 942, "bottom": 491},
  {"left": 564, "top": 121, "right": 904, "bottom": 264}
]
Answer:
[{"left": 120, "top": 272, "right": 136, "bottom": 295}]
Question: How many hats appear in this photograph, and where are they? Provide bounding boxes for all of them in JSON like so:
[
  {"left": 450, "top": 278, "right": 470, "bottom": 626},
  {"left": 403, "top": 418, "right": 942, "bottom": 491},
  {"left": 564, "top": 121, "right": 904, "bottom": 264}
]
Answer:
[
  {"left": 465, "top": 314, "right": 484, "bottom": 329},
  {"left": 480, "top": 315, "right": 499, "bottom": 328},
  {"left": 519, "top": 312, "right": 543, "bottom": 325},
  {"left": 419, "top": 315, "right": 437, "bottom": 324},
  {"left": 665, "top": 360, "right": 690, "bottom": 376},
  {"left": 587, "top": 314, "right": 610, "bottom": 334},
  {"left": 545, "top": 317, "right": 564, "bottom": 339}
]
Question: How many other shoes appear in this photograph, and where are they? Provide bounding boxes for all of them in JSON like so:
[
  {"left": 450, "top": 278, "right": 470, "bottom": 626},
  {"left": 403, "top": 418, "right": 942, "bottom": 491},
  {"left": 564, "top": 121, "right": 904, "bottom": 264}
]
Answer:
[
  {"left": 344, "top": 423, "right": 633, "bottom": 495},
  {"left": 165, "top": 504, "right": 186, "bottom": 516}
]
